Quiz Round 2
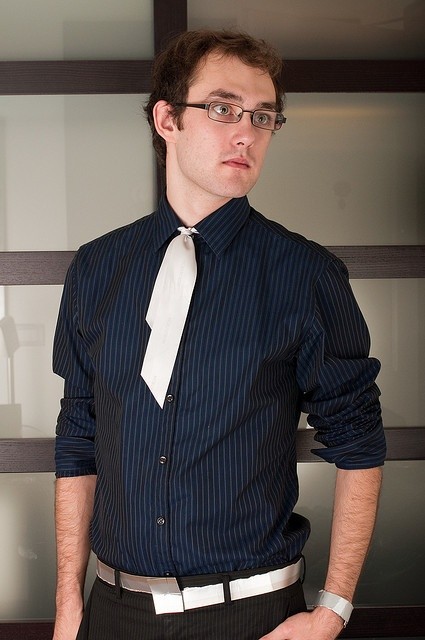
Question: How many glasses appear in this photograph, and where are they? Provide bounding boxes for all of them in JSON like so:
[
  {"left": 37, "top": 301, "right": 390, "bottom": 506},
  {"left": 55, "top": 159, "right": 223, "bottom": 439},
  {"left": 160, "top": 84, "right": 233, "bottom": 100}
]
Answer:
[{"left": 173, "top": 100, "right": 287, "bottom": 131}]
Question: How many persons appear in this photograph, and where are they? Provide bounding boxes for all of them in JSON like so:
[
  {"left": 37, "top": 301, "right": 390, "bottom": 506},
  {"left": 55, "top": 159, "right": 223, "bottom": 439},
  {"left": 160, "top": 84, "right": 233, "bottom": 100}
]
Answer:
[{"left": 52, "top": 29, "right": 386, "bottom": 640}]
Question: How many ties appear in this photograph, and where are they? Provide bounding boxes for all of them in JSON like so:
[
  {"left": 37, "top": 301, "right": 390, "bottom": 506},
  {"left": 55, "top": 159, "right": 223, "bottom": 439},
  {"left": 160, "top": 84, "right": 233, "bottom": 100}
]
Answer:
[{"left": 139, "top": 226, "right": 200, "bottom": 422}]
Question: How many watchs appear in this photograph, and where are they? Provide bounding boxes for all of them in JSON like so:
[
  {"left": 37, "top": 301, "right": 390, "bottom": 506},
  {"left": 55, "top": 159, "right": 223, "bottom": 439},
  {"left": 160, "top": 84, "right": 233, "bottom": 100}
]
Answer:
[{"left": 312, "top": 589, "right": 354, "bottom": 628}]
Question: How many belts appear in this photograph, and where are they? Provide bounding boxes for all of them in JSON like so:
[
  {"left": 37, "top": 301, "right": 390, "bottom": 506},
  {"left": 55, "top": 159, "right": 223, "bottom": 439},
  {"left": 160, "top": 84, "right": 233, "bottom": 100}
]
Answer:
[{"left": 97, "top": 558, "right": 304, "bottom": 615}]
